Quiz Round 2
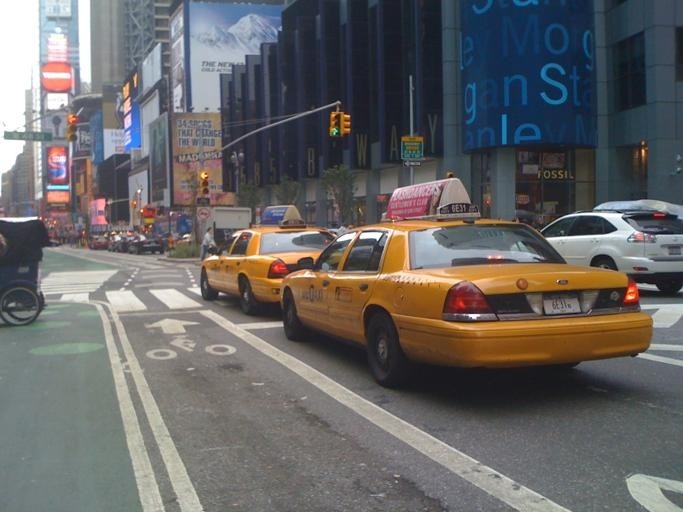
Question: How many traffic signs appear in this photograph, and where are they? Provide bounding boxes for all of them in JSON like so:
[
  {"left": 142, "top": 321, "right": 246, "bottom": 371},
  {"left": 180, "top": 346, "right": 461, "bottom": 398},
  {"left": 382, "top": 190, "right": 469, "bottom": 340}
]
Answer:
[{"left": 398, "top": 134, "right": 428, "bottom": 166}]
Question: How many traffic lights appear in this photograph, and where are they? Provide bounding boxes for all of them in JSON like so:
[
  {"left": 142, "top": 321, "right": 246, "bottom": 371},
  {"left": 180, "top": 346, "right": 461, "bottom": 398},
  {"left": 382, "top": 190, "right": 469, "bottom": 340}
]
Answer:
[
  {"left": 327, "top": 111, "right": 351, "bottom": 138},
  {"left": 65, "top": 113, "right": 78, "bottom": 143},
  {"left": 199, "top": 169, "right": 209, "bottom": 196}
]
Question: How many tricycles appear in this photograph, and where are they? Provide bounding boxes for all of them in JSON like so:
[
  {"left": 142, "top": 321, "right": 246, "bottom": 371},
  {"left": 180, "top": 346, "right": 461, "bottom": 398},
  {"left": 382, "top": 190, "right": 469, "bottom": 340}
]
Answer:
[{"left": 0, "top": 215, "right": 50, "bottom": 327}]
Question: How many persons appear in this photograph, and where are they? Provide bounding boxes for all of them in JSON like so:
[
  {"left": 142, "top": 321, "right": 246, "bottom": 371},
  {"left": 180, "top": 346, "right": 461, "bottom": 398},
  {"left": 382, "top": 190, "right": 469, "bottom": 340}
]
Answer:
[{"left": 200, "top": 227, "right": 217, "bottom": 262}]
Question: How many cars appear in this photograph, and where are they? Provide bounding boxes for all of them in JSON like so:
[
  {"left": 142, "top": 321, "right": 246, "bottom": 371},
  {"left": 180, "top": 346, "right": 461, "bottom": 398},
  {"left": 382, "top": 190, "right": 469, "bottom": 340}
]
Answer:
[
  {"left": 281, "top": 176, "right": 654, "bottom": 388},
  {"left": 536, "top": 210, "right": 682, "bottom": 296},
  {"left": 200, "top": 204, "right": 348, "bottom": 315},
  {"left": 86, "top": 228, "right": 165, "bottom": 255}
]
